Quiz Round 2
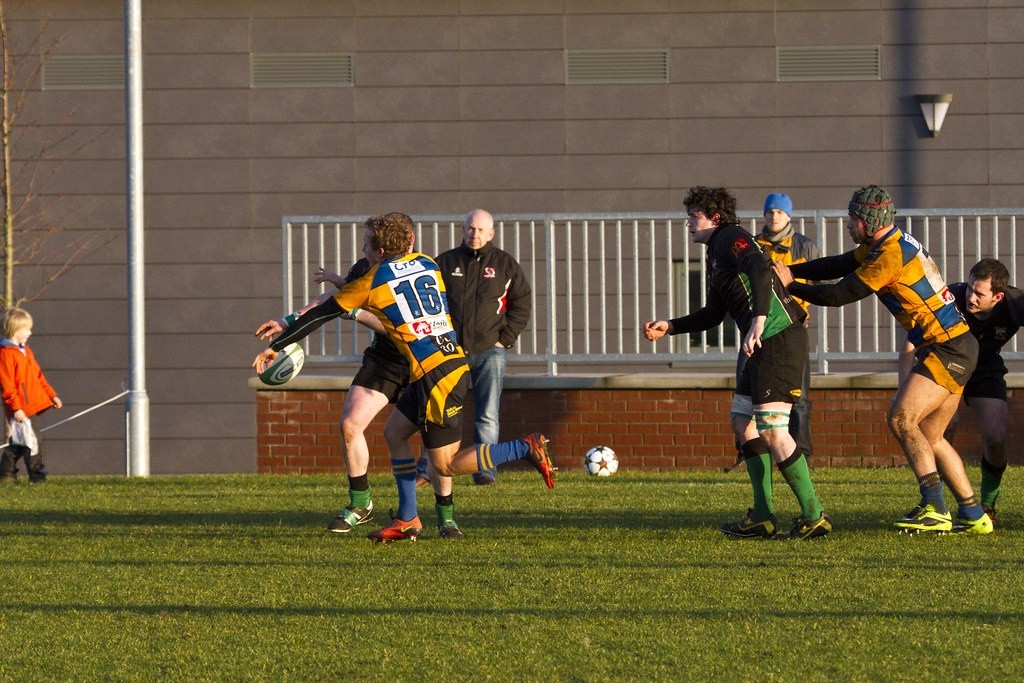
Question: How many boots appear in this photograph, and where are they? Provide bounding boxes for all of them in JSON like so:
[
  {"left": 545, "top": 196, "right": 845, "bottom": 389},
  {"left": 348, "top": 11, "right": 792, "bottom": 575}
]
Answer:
[
  {"left": 23, "top": 446, "right": 46, "bottom": 483},
  {"left": 0, "top": 436, "right": 24, "bottom": 483}
]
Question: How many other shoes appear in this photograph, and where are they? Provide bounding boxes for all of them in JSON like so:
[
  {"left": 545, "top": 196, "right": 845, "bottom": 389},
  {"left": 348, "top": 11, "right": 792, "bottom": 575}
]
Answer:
[
  {"left": 475, "top": 476, "right": 494, "bottom": 484},
  {"left": 415, "top": 478, "right": 428, "bottom": 490}
]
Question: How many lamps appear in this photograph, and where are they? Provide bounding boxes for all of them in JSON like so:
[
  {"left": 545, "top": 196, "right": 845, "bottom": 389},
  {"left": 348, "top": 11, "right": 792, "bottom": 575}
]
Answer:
[{"left": 915, "top": 94, "right": 953, "bottom": 137}]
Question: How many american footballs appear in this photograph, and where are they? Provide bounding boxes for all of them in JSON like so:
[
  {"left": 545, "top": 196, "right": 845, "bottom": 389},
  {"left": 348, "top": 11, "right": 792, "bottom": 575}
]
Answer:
[{"left": 257, "top": 341, "right": 305, "bottom": 386}]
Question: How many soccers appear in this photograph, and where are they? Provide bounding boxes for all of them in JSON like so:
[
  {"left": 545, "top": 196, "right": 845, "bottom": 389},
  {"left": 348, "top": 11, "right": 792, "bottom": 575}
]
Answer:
[{"left": 584, "top": 446, "right": 619, "bottom": 478}]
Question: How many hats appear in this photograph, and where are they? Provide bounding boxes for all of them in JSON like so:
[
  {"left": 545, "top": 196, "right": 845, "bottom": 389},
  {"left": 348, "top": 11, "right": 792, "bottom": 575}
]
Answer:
[
  {"left": 847, "top": 184, "right": 896, "bottom": 246},
  {"left": 764, "top": 193, "right": 792, "bottom": 217}
]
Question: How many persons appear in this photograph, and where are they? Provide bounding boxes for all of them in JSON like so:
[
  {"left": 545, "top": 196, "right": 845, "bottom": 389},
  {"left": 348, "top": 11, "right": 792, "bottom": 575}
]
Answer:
[
  {"left": 408, "top": 209, "right": 532, "bottom": 487},
  {"left": 770, "top": 184, "right": 993, "bottom": 536},
  {"left": 252, "top": 213, "right": 559, "bottom": 543},
  {"left": 890, "top": 258, "right": 1024, "bottom": 529},
  {"left": 644, "top": 186, "right": 832, "bottom": 539},
  {"left": 0, "top": 309, "right": 62, "bottom": 483},
  {"left": 724, "top": 192, "right": 834, "bottom": 473}
]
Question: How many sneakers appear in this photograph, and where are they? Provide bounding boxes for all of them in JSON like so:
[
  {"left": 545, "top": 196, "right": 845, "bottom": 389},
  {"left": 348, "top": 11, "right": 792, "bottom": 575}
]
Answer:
[
  {"left": 787, "top": 511, "right": 833, "bottom": 541},
  {"left": 902, "top": 505, "right": 924, "bottom": 520},
  {"left": 938, "top": 513, "right": 993, "bottom": 536},
  {"left": 982, "top": 506, "right": 996, "bottom": 523},
  {"left": 330, "top": 499, "right": 374, "bottom": 532},
  {"left": 892, "top": 503, "right": 952, "bottom": 536},
  {"left": 720, "top": 513, "right": 778, "bottom": 539},
  {"left": 437, "top": 518, "right": 460, "bottom": 539},
  {"left": 522, "top": 432, "right": 558, "bottom": 489},
  {"left": 367, "top": 508, "right": 422, "bottom": 542}
]
